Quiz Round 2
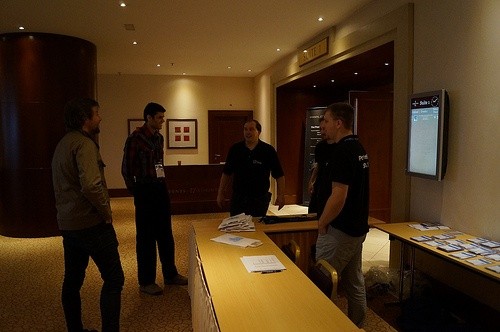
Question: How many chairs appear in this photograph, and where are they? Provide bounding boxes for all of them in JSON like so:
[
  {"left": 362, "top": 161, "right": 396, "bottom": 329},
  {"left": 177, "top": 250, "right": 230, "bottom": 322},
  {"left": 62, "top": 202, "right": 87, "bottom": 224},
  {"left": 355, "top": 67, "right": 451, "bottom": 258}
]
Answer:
[
  {"left": 282, "top": 240, "right": 300, "bottom": 263},
  {"left": 307, "top": 260, "right": 339, "bottom": 301}
]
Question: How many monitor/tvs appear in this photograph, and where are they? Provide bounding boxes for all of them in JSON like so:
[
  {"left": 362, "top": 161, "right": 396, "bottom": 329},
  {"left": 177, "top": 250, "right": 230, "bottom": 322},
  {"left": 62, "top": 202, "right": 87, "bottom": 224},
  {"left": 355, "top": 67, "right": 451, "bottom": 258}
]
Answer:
[{"left": 404, "top": 88, "right": 449, "bottom": 182}]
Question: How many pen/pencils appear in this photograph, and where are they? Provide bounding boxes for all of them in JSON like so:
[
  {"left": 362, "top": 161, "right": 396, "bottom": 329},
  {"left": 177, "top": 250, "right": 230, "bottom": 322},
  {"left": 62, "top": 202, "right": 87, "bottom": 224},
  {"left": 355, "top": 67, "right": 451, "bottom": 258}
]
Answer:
[{"left": 262, "top": 270, "right": 283, "bottom": 274}]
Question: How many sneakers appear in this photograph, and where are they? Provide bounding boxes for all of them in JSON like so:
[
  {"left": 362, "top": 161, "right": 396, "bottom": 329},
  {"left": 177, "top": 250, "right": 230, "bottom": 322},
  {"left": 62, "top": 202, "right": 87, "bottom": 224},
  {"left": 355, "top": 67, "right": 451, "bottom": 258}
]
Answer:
[
  {"left": 140, "top": 283, "right": 163, "bottom": 295},
  {"left": 168, "top": 274, "right": 188, "bottom": 284}
]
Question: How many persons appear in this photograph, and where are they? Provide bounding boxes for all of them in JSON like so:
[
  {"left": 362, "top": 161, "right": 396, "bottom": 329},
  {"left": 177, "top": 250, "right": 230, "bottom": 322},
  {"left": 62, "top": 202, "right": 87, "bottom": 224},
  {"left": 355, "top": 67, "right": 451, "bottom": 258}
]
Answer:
[
  {"left": 53, "top": 98, "right": 125, "bottom": 332},
  {"left": 216, "top": 119, "right": 285, "bottom": 216},
  {"left": 121, "top": 102, "right": 188, "bottom": 294},
  {"left": 308, "top": 116, "right": 328, "bottom": 219},
  {"left": 315, "top": 102, "right": 369, "bottom": 329}
]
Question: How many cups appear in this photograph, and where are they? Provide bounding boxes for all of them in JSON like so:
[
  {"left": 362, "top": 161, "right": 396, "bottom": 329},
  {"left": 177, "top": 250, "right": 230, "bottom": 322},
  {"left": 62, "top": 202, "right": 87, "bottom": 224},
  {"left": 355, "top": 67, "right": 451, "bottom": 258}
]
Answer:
[{"left": 178, "top": 161, "right": 181, "bottom": 165}]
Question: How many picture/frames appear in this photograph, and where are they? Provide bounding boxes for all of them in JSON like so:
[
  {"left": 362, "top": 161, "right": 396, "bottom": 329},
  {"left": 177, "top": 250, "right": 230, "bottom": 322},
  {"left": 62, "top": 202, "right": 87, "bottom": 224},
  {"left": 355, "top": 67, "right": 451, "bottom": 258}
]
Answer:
[
  {"left": 166, "top": 119, "right": 198, "bottom": 149},
  {"left": 128, "top": 119, "right": 145, "bottom": 136}
]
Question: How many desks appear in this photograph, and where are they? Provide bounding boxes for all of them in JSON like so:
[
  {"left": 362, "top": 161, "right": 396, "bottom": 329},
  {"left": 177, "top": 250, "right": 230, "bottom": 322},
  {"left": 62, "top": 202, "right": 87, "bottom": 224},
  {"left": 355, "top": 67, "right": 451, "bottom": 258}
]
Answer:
[
  {"left": 165, "top": 165, "right": 230, "bottom": 215},
  {"left": 188, "top": 212, "right": 384, "bottom": 332},
  {"left": 375, "top": 220, "right": 500, "bottom": 301}
]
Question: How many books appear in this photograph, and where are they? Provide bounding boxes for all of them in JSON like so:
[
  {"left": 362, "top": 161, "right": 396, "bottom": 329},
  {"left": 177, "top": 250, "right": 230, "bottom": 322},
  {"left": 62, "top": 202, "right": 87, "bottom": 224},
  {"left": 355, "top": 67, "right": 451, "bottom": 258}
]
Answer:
[
  {"left": 424, "top": 239, "right": 496, "bottom": 255},
  {"left": 449, "top": 251, "right": 477, "bottom": 259},
  {"left": 465, "top": 258, "right": 493, "bottom": 265},
  {"left": 485, "top": 254, "right": 500, "bottom": 261},
  {"left": 485, "top": 264, "right": 500, "bottom": 274},
  {"left": 434, "top": 233, "right": 457, "bottom": 239},
  {"left": 218, "top": 213, "right": 257, "bottom": 231},
  {"left": 466, "top": 236, "right": 491, "bottom": 244},
  {"left": 410, "top": 234, "right": 433, "bottom": 242},
  {"left": 481, "top": 241, "right": 500, "bottom": 247}
]
